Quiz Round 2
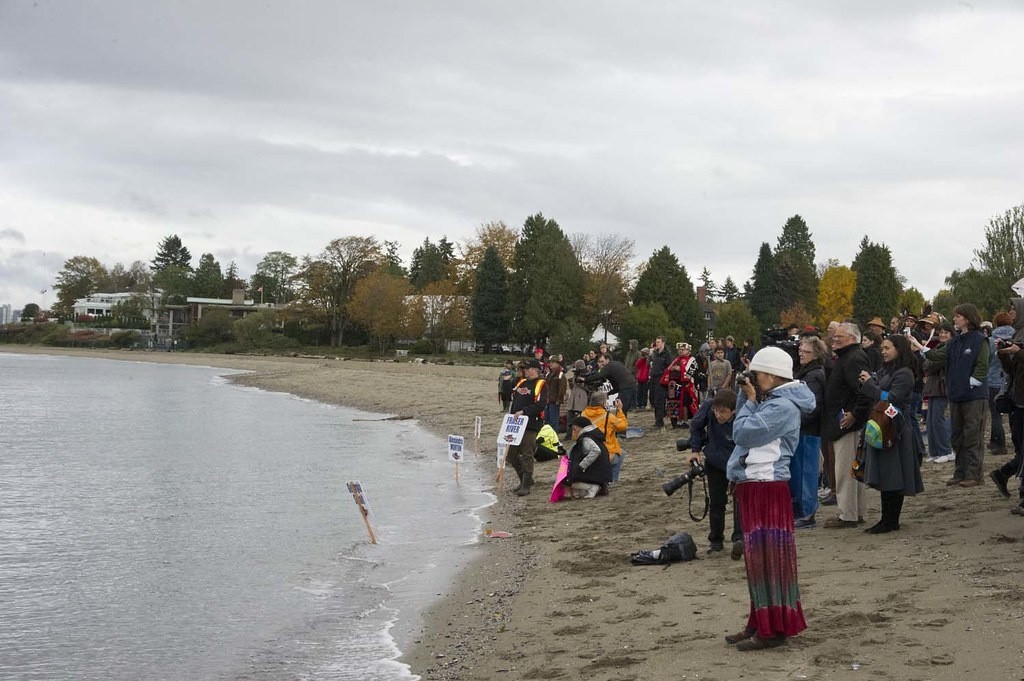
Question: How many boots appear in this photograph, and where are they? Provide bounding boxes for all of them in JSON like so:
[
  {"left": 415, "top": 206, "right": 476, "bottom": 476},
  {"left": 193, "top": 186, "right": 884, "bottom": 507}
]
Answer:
[
  {"left": 513, "top": 470, "right": 526, "bottom": 492},
  {"left": 517, "top": 471, "right": 533, "bottom": 495}
]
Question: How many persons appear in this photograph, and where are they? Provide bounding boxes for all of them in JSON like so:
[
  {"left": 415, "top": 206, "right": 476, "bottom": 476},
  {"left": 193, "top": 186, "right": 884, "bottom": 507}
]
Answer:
[
  {"left": 499, "top": 304, "right": 1024, "bottom": 560},
  {"left": 725, "top": 345, "right": 817, "bottom": 652}
]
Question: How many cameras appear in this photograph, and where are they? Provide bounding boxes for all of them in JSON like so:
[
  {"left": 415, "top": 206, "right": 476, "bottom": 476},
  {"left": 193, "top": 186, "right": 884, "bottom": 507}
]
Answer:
[
  {"left": 998, "top": 339, "right": 1011, "bottom": 348},
  {"left": 903, "top": 327, "right": 910, "bottom": 336},
  {"left": 737, "top": 371, "right": 757, "bottom": 385},
  {"left": 676, "top": 436, "right": 708, "bottom": 451},
  {"left": 613, "top": 401, "right": 615, "bottom": 405},
  {"left": 662, "top": 459, "right": 706, "bottom": 497}
]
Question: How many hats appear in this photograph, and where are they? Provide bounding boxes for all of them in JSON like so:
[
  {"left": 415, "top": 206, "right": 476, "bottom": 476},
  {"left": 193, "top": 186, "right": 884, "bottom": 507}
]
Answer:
[
  {"left": 505, "top": 360, "right": 513, "bottom": 365},
  {"left": 749, "top": 345, "right": 794, "bottom": 380},
  {"left": 519, "top": 360, "right": 541, "bottom": 369},
  {"left": 865, "top": 316, "right": 886, "bottom": 329},
  {"left": 640, "top": 347, "right": 651, "bottom": 354},
  {"left": 921, "top": 315, "right": 940, "bottom": 325},
  {"left": 726, "top": 335, "right": 734, "bottom": 340},
  {"left": 535, "top": 348, "right": 543, "bottom": 353},
  {"left": 547, "top": 354, "right": 561, "bottom": 362},
  {"left": 570, "top": 360, "right": 587, "bottom": 370},
  {"left": 568, "top": 417, "right": 593, "bottom": 429}
]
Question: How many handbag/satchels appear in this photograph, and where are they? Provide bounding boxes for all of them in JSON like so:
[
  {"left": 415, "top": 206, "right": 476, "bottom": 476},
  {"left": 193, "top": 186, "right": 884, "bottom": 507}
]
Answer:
[
  {"left": 864, "top": 397, "right": 906, "bottom": 449},
  {"left": 628, "top": 531, "right": 695, "bottom": 567}
]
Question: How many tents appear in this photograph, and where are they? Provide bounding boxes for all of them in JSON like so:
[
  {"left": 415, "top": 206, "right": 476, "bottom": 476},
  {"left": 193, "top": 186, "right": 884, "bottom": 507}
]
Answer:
[{"left": 580, "top": 323, "right": 622, "bottom": 351}]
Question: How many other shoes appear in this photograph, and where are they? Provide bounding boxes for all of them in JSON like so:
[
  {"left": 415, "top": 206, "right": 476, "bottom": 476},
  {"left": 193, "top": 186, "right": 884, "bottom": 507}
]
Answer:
[
  {"left": 724, "top": 623, "right": 753, "bottom": 642},
  {"left": 736, "top": 628, "right": 786, "bottom": 649},
  {"left": 708, "top": 436, "right": 1020, "bottom": 561}
]
